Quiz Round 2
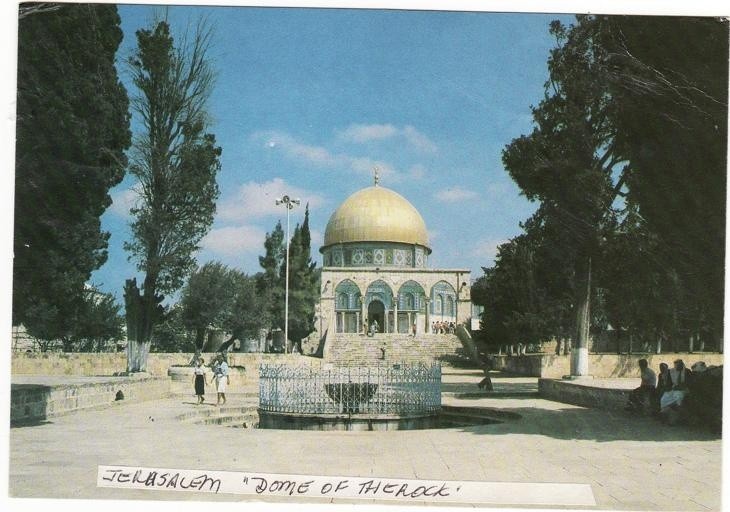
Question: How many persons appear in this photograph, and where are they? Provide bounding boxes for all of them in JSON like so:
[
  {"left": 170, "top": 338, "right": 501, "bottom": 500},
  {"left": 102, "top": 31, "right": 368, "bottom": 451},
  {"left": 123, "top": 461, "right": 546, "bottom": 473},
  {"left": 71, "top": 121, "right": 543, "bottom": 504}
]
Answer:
[
  {"left": 370, "top": 323, "right": 376, "bottom": 337},
  {"left": 191, "top": 358, "right": 208, "bottom": 405},
  {"left": 373, "top": 320, "right": 379, "bottom": 332},
  {"left": 478, "top": 358, "right": 492, "bottom": 391},
  {"left": 382, "top": 342, "right": 387, "bottom": 360},
  {"left": 210, "top": 355, "right": 230, "bottom": 407},
  {"left": 412, "top": 324, "right": 417, "bottom": 337},
  {"left": 431, "top": 321, "right": 456, "bottom": 335},
  {"left": 362, "top": 319, "right": 368, "bottom": 335},
  {"left": 624, "top": 359, "right": 723, "bottom": 435}
]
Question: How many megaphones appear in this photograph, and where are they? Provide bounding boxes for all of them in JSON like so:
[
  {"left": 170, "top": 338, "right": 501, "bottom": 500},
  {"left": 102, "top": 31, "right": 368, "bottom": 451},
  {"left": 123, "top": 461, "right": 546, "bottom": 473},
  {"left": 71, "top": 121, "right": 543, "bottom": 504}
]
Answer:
[
  {"left": 283, "top": 195, "right": 290, "bottom": 203},
  {"left": 276, "top": 199, "right": 284, "bottom": 205},
  {"left": 291, "top": 197, "right": 301, "bottom": 207},
  {"left": 286, "top": 202, "right": 293, "bottom": 209}
]
